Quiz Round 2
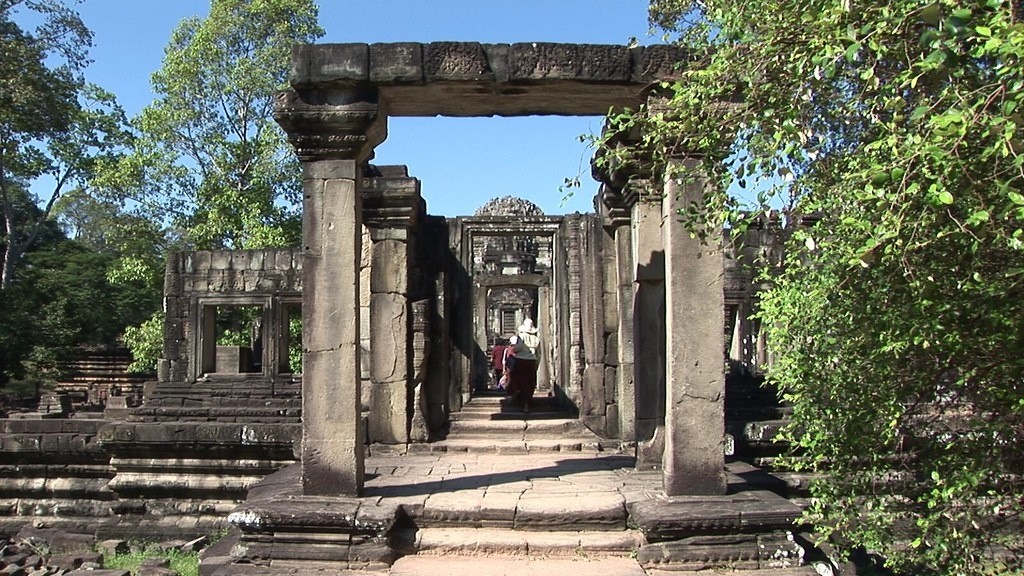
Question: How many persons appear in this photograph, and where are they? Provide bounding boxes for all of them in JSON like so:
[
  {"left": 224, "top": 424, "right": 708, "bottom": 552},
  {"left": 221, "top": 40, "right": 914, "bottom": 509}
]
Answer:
[
  {"left": 491, "top": 338, "right": 507, "bottom": 392},
  {"left": 501, "top": 317, "right": 542, "bottom": 412}
]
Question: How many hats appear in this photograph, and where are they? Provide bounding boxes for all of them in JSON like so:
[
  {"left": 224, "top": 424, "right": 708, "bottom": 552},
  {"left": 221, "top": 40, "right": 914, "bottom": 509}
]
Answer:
[
  {"left": 518, "top": 318, "right": 538, "bottom": 333},
  {"left": 510, "top": 336, "right": 517, "bottom": 344}
]
